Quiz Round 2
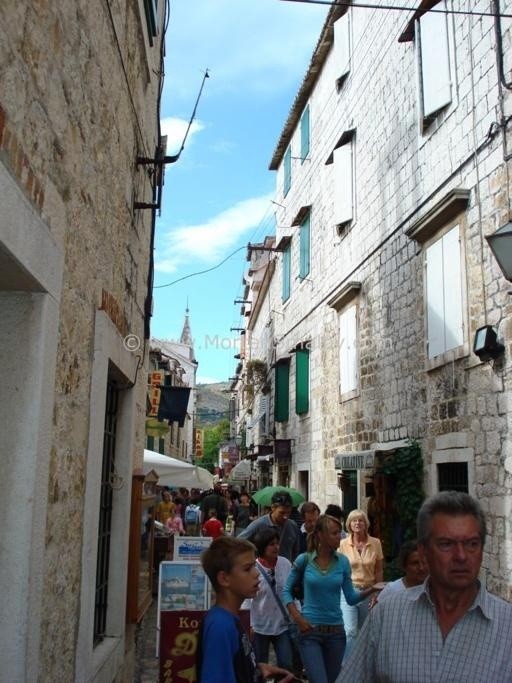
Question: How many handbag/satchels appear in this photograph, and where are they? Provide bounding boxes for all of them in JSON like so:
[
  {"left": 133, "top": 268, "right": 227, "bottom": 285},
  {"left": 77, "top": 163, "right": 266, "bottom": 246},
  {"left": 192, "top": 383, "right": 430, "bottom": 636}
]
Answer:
[
  {"left": 293, "top": 553, "right": 308, "bottom": 598},
  {"left": 288, "top": 623, "right": 297, "bottom": 644}
]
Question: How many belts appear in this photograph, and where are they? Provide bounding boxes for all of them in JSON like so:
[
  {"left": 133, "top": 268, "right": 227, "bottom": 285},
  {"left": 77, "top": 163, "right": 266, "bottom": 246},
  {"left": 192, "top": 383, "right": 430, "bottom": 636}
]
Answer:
[{"left": 313, "top": 624, "right": 344, "bottom": 631}]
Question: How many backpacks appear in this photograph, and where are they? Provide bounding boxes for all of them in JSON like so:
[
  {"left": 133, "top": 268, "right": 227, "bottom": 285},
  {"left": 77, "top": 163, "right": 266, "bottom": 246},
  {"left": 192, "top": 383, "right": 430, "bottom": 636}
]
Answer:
[{"left": 186, "top": 511, "right": 197, "bottom": 525}]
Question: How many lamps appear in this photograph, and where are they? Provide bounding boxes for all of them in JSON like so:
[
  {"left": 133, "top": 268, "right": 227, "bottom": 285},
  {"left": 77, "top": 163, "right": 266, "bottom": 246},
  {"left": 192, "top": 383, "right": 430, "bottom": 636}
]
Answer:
[
  {"left": 484, "top": 217, "right": 512, "bottom": 296},
  {"left": 473, "top": 325, "right": 504, "bottom": 363}
]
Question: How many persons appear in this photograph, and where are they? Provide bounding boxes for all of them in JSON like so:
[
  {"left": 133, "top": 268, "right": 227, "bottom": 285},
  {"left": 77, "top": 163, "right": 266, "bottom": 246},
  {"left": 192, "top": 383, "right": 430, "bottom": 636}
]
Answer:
[{"left": 156, "top": 480, "right": 512, "bottom": 683}]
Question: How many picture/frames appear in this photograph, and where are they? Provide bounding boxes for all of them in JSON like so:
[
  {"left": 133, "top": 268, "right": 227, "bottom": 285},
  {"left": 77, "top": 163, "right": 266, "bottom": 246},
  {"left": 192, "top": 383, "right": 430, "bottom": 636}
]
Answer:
[
  {"left": 155, "top": 561, "right": 211, "bottom": 658},
  {"left": 173, "top": 536, "right": 213, "bottom": 561}
]
